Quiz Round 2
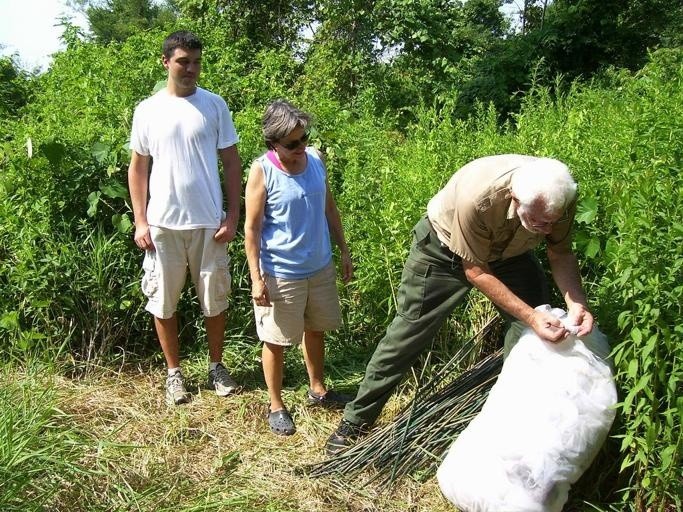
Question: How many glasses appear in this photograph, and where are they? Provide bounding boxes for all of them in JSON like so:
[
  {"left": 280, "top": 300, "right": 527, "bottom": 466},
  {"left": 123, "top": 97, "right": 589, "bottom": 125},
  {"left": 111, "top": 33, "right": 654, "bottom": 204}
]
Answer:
[
  {"left": 278, "top": 128, "right": 311, "bottom": 150},
  {"left": 527, "top": 210, "right": 570, "bottom": 229}
]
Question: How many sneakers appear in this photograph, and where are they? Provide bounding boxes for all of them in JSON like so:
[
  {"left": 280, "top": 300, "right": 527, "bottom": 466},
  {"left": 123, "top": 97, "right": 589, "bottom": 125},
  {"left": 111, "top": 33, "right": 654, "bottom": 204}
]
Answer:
[
  {"left": 207, "top": 363, "right": 239, "bottom": 397},
  {"left": 327, "top": 417, "right": 367, "bottom": 455},
  {"left": 166, "top": 370, "right": 189, "bottom": 404}
]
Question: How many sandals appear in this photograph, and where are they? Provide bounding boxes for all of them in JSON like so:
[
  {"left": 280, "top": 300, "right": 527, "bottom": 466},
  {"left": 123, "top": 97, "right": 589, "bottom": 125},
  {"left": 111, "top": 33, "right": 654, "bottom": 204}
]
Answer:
[
  {"left": 307, "top": 385, "right": 355, "bottom": 404},
  {"left": 267, "top": 402, "right": 295, "bottom": 436}
]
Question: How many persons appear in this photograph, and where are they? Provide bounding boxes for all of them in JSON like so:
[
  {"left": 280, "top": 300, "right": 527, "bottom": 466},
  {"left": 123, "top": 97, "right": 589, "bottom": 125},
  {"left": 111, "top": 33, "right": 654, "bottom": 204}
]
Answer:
[
  {"left": 242, "top": 97, "right": 355, "bottom": 437},
  {"left": 125, "top": 29, "right": 242, "bottom": 407},
  {"left": 322, "top": 153, "right": 595, "bottom": 458}
]
